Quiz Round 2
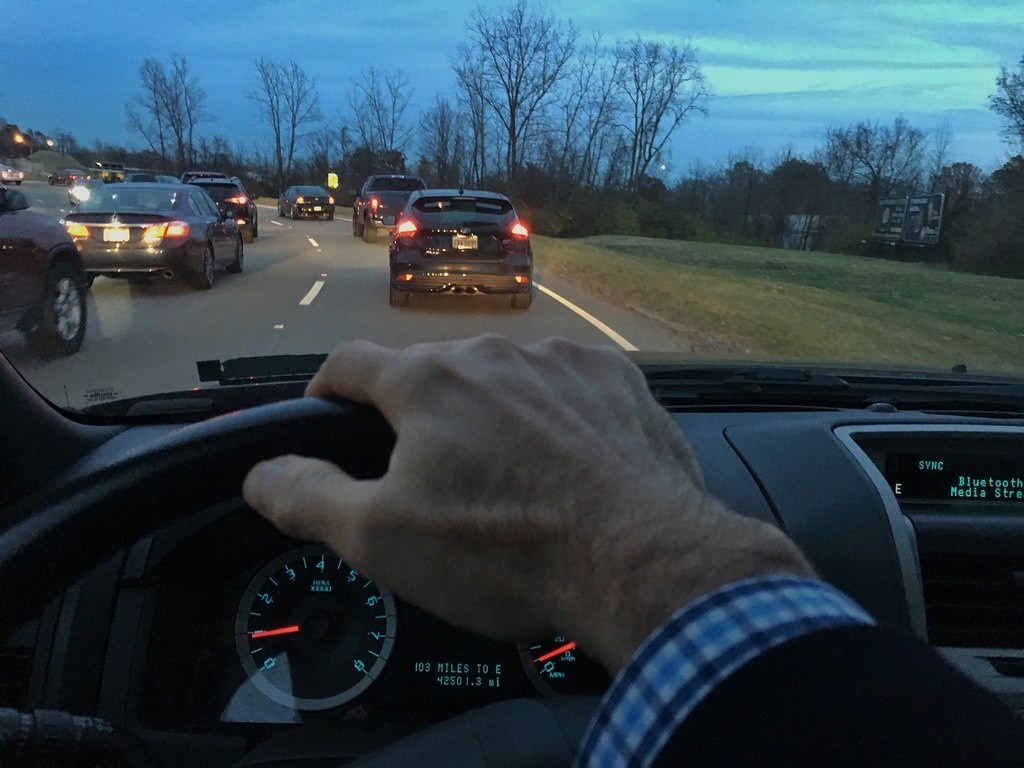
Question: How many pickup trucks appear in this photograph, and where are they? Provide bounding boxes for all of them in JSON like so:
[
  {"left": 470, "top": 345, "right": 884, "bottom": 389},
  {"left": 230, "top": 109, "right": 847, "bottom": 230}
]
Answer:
[{"left": 353, "top": 174, "right": 429, "bottom": 244}]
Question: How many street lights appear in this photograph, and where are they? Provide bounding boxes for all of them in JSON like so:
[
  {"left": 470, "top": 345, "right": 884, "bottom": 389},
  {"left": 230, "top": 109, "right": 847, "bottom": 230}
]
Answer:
[{"left": 17, "top": 137, "right": 33, "bottom": 173}]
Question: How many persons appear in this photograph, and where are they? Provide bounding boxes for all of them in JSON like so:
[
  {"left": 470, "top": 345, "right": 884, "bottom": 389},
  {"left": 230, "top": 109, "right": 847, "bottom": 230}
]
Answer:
[{"left": 242, "top": 333, "right": 1024, "bottom": 768}]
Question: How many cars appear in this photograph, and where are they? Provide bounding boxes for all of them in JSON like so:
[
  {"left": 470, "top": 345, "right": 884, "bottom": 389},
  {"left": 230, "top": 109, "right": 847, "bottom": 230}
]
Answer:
[
  {"left": 389, "top": 189, "right": 534, "bottom": 308},
  {"left": 58, "top": 182, "right": 244, "bottom": 289},
  {"left": 122, "top": 171, "right": 231, "bottom": 184},
  {"left": 0, "top": 181, "right": 86, "bottom": 356},
  {"left": 48, "top": 169, "right": 92, "bottom": 187},
  {"left": 278, "top": 186, "right": 335, "bottom": 221},
  {"left": 0, "top": 165, "right": 24, "bottom": 185}
]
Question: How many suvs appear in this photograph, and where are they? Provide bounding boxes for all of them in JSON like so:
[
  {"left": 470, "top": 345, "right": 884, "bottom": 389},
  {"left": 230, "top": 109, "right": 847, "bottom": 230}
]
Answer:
[{"left": 173, "top": 176, "right": 258, "bottom": 244}]
